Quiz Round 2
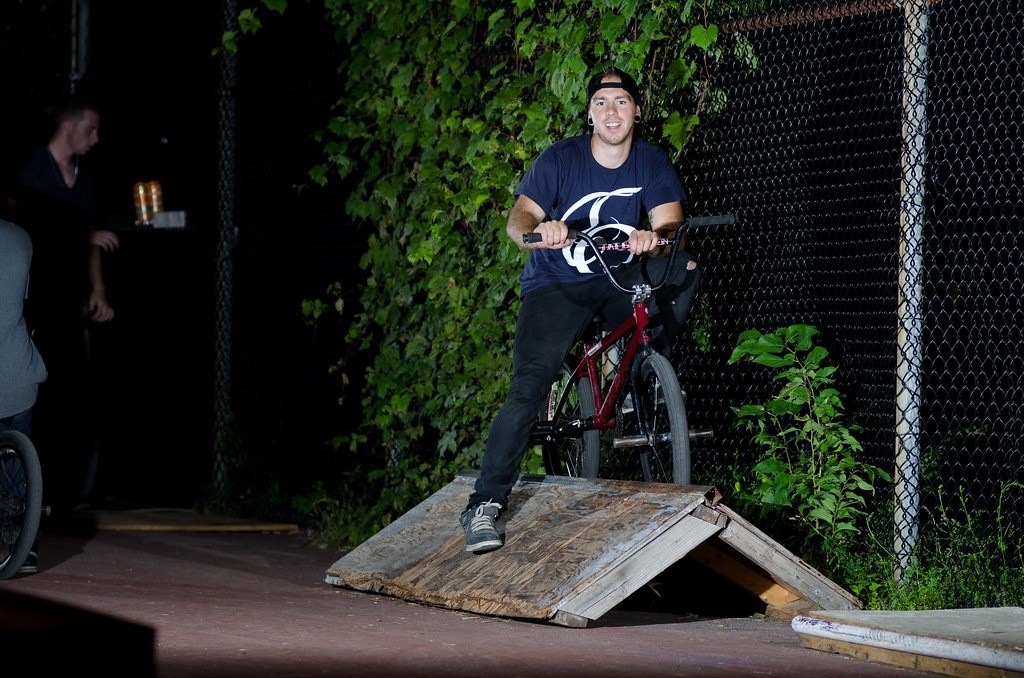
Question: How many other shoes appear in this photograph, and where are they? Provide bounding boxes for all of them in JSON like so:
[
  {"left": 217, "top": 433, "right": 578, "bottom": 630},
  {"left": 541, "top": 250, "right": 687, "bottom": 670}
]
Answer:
[{"left": 0, "top": 537, "right": 39, "bottom": 574}]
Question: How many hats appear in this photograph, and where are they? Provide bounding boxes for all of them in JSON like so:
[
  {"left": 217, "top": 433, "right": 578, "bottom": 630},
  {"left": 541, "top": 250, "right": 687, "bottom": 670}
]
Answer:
[{"left": 586, "top": 65, "right": 641, "bottom": 110}]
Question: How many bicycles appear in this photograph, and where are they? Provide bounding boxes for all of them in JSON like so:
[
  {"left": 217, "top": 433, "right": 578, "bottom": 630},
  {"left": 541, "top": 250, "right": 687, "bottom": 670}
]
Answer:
[
  {"left": 522, "top": 213, "right": 715, "bottom": 488},
  {"left": 0, "top": 431, "right": 43, "bottom": 581}
]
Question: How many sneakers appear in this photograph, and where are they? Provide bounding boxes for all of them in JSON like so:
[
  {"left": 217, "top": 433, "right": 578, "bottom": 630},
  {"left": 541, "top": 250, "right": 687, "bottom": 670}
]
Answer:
[
  {"left": 458, "top": 498, "right": 503, "bottom": 552},
  {"left": 621, "top": 384, "right": 686, "bottom": 414}
]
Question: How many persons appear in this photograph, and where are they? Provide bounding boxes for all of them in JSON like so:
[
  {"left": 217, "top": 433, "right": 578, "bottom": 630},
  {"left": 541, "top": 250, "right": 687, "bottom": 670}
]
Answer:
[
  {"left": 458, "top": 66, "right": 701, "bottom": 554},
  {"left": 0, "top": 101, "right": 120, "bottom": 517},
  {"left": 0, "top": 221, "right": 50, "bottom": 575}
]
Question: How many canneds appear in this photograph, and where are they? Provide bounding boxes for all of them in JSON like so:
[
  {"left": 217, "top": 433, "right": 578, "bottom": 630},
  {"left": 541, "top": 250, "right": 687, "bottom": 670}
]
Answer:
[{"left": 132, "top": 179, "right": 162, "bottom": 224}]
